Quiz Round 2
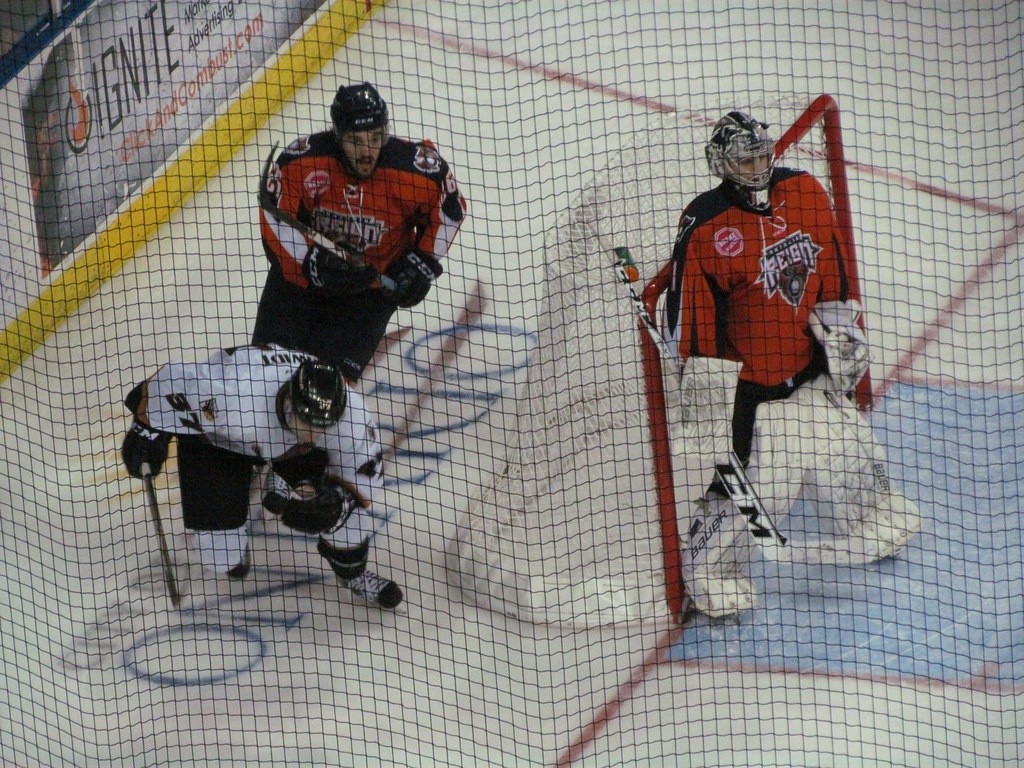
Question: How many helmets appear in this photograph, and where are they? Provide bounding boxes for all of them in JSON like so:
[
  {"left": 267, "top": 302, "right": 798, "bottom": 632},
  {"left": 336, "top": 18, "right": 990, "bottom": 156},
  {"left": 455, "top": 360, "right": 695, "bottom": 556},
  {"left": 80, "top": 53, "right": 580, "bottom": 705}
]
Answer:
[
  {"left": 330, "top": 82, "right": 386, "bottom": 131},
  {"left": 290, "top": 360, "right": 347, "bottom": 428},
  {"left": 705, "top": 112, "right": 774, "bottom": 179}
]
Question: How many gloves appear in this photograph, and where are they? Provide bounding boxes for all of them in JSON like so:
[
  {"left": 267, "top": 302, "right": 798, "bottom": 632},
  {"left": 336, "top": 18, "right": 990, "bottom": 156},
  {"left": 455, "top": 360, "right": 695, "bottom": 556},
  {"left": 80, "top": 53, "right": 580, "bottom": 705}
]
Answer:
[
  {"left": 121, "top": 420, "right": 172, "bottom": 478},
  {"left": 388, "top": 249, "right": 443, "bottom": 307},
  {"left": 280, "top": 489, "right": 351, "bottom": 534},
  {"left": 357, "top": 274, "right": 397, "bottom": 318}
]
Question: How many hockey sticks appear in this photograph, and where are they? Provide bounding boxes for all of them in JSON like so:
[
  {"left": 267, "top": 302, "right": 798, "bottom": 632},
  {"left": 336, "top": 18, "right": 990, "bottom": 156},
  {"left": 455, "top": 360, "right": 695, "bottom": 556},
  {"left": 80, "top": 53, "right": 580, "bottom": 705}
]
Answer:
[
  {"left": 258, "top": 140, "right": 398, "bottom": 292},
  {"left": 142, "top": 463, "right": 193, "bottom": 610},
  {"left": 586, "top": 216, "right": 876, "bottom": 566}
]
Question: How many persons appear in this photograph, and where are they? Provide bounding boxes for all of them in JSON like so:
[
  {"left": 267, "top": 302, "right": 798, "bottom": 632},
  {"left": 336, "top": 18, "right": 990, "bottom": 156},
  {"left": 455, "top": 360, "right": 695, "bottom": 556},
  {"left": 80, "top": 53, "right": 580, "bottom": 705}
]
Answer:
[
  {"left": 121, "top": 340, "right": 405, "bottom": 612},
  {"left": 250, "top": 80, "right": 468, "bottom": 384},
  {"left": 670, "top": 111, "right": 922, "bottom": 623}
]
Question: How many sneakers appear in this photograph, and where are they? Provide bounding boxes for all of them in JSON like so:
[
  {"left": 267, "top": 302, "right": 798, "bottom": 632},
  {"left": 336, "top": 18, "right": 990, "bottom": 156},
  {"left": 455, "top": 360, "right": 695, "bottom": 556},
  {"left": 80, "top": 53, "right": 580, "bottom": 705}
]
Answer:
[
  {"left": 261, "top": 466, "right": 292, "bottom": 521},
  {"left": 225, "top": 541, "right": 252, "bottom": 581},
  {"left": 337, "top": 569, "right": 405, "bottom": 613}
]
